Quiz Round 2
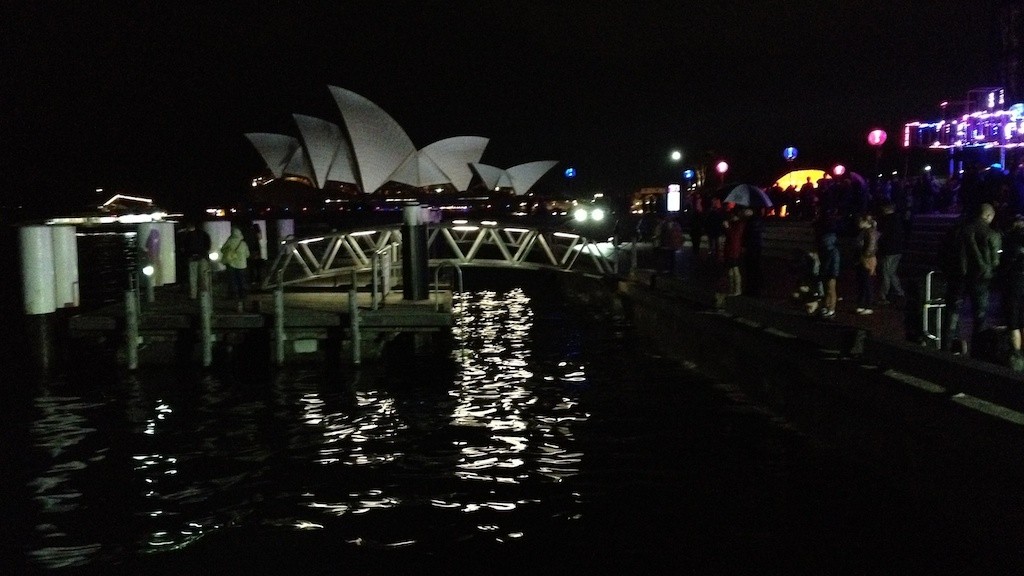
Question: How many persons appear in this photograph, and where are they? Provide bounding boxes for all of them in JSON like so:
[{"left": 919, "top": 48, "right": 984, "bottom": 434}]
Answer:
[
  {"left": 221, "top": 221, "right": 262, "bottom": 313},
  {"left": 181, "top": 224, "right": 212, "bottom": 299},
  {"left": 611, "top": 153, "right": 1024, "bottom": 376}
]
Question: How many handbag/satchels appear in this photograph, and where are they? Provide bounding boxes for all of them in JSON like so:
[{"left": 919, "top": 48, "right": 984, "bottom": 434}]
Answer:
[{"left": 221, "top": 251, "right": 234, "bottom": 265}]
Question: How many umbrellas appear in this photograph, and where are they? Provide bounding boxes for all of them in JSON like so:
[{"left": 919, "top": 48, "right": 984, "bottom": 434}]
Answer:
[{"left": 723, "top": 183, "right": 773, "bottom": 211}]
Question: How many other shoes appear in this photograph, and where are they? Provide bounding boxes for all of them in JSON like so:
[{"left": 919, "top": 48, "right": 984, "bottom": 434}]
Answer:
[
  {"left": 824, "top": 310, "right": 835, "bottom": 317},
  {"left": 862, "top": 309, "right": 874, "bottom": 314},
  {"left": 857, "top": 307, "right": 865, "bottom": 312}
]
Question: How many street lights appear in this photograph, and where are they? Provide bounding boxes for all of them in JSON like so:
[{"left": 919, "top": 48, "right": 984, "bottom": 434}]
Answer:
[
  {"left": 716, "top": 161, "right": 728, "bottom": 187},
  {"left": 867, "top": 128, "right": 887, "bottom": 174},
  {"left": 669, "top": 149, "right": 682, "bottom": 183}
]
